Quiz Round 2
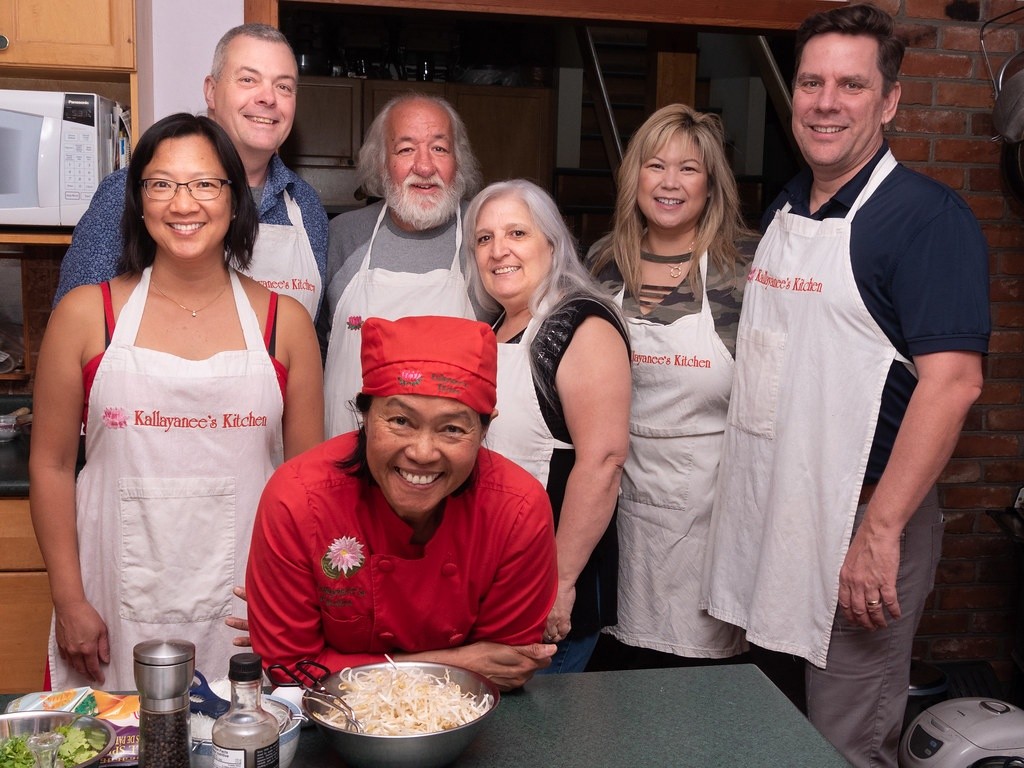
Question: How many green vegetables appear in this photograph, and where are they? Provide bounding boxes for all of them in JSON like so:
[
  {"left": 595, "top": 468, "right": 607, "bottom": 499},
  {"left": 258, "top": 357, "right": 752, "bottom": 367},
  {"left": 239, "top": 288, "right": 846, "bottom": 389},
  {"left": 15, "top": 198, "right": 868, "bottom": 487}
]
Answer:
[{"left": 0, "top": 726, "right": 101, "bottom": 768}]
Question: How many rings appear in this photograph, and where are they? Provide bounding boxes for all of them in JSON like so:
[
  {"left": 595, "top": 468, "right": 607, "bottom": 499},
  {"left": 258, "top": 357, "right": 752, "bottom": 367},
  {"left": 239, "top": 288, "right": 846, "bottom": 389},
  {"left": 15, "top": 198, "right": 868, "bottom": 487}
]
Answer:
[{"left": 866, "top": 600, "right": 881, "bottom": 606}]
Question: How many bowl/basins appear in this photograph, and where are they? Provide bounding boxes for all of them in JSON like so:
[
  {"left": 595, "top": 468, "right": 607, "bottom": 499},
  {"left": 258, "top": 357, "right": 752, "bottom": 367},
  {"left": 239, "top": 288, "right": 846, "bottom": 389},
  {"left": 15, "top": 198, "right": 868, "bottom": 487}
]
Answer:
[
  {"left": 296, "top": 53, "right": 319, "bottom": 75},
  {"left": 0, "top": 710, "right": 117, "bottom": 768},
  {"left": 189, "top": 694, "right": 301, "bottom": 767},
  {"left": 301, "top": 661, "right": 500, "bottom": 768},
  {"left": 0, "top": 415, "right": 19, "bottom": 442}
]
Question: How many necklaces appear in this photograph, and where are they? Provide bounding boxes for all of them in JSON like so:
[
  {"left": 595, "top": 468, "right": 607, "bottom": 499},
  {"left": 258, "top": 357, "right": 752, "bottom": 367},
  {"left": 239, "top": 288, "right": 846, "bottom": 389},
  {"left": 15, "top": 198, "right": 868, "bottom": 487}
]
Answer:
[
  {"left": 151, "top": 279, "right": 230, "bottom": 317},
  {"left": 645, "top": 237, "right": 695, "bottom": 278}
]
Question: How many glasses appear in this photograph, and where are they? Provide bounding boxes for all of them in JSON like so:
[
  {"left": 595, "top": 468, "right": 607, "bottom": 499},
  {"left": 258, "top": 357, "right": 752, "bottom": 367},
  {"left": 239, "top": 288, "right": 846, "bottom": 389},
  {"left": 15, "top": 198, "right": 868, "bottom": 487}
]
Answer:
[{"left": 139, "top": 178, "right": 231, "bottom": 201}]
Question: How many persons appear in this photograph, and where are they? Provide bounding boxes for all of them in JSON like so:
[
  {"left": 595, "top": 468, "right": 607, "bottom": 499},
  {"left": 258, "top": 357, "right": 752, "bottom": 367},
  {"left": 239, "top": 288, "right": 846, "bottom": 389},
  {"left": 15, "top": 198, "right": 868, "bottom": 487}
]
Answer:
[
  {"left": 462, "top": 178, "right": 632, "bottom": 675},
  {"left": 323, "top": 94, "right": 503, "bottom": 440},
  {"left": 697, "top": 3, "right": 993, "bottom": 768},
  {"left": 582, "top": 103, "right": 790, "bottom": 699},
  {"left": 27, "top": 112, "right": 326, "bottom": 692},
  {"left": 224, "top": 315, "right": 558, "bottom": 692},
  {"left": 51, "top": 22, "right": 329, "bottom": 356}
]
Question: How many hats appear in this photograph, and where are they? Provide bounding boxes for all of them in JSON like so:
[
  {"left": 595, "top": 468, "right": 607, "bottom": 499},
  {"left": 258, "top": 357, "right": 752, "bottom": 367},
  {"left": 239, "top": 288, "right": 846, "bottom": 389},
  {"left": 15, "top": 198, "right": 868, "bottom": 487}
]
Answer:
[{"left": 360, "top": 315, "right": 497, "bottom": 415}]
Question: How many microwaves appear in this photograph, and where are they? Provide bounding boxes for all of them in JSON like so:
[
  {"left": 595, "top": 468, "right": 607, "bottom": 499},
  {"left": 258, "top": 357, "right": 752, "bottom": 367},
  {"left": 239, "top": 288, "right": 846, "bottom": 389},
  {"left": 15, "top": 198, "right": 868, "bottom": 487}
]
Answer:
[{"left": 0, "top": 89, "right": 116, "bottom": 226}]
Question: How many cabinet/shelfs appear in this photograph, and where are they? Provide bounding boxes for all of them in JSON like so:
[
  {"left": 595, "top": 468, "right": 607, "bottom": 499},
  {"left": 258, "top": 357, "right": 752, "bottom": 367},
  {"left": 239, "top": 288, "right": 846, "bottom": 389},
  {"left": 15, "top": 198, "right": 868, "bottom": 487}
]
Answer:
[
  {"left": 245, "top": 77, "right": 551, "bottom": 212},
  {"left": 0, "top": 1, "right": 140, "bottom": 701}
]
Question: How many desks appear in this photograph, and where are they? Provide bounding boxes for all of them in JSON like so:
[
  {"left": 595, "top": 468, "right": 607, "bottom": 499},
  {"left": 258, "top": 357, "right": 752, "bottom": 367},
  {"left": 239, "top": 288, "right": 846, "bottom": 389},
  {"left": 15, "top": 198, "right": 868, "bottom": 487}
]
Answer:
[{"left": 0, "top": 664, "right": 856, "bottom": 768}]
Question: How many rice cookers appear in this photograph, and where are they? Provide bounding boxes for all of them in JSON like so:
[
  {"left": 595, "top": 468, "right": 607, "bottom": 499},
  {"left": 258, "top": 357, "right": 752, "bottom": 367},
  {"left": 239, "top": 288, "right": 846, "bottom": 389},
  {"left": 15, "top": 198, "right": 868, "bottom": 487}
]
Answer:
[{"left": 900, "top": 696, "right": 1024, "bottom": 768}]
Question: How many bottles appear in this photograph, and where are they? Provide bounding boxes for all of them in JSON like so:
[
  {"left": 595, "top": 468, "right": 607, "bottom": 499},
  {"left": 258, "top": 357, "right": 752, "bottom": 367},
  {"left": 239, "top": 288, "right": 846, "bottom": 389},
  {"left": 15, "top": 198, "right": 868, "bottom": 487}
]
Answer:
[
  {"left": 133, "top": 638, "right": 196, "bottom": 768},
  {"left": 211, "top": 653, "right": 280, "bottom": 768},
  {"left": 24, "top": 733, "right": 65, "bottom": 768}
]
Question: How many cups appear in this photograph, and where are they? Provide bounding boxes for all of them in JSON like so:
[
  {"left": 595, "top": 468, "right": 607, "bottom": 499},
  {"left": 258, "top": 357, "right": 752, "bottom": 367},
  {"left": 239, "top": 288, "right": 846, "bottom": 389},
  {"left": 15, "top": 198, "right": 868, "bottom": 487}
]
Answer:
[{"left": 416, "top": 59, "right": 434, "bottom": 81}]
